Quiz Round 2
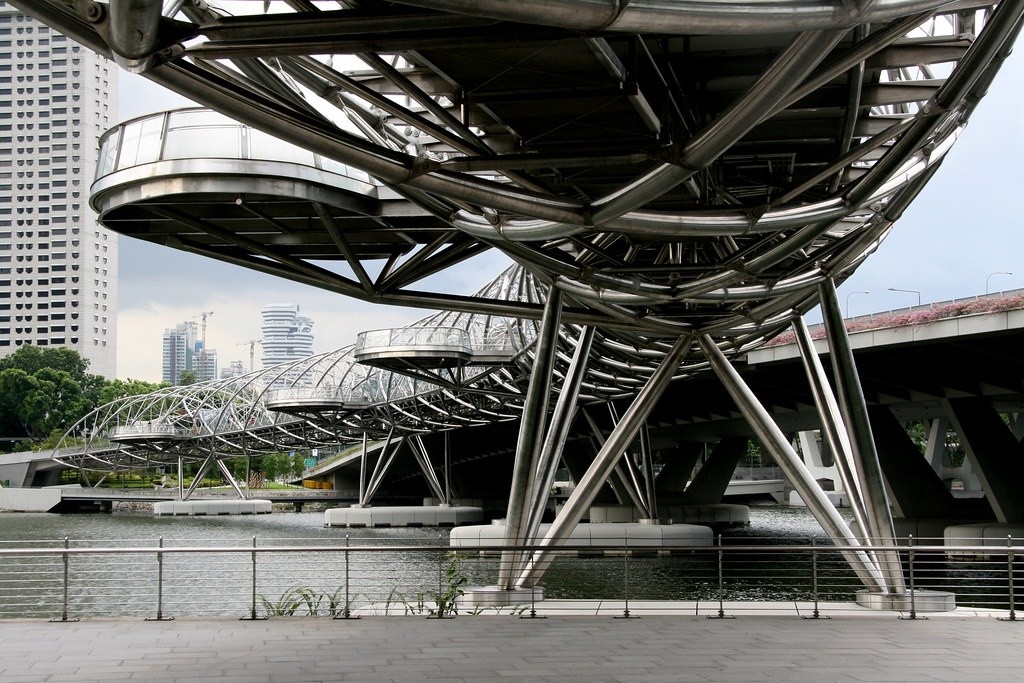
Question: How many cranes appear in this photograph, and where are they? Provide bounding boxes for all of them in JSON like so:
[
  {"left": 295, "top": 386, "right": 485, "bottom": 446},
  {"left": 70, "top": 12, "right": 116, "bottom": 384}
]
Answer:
[
  {"left": 191, "top": 311, "right": 215, "bottom": 382},
  {"left": 236, "top": 338, "right": 262, "bottom": 372}
]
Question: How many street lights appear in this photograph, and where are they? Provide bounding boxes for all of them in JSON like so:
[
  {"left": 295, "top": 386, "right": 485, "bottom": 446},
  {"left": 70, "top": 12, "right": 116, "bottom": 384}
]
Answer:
[
  {"left": 846, "top": 291, "right": 870, "bottom": 318},
  {"left": 888, "top": 288, "right": 921, "bottom": 305},
  {"left": 986, "top": 272, "right": 1013, "bottom": 294}
]
